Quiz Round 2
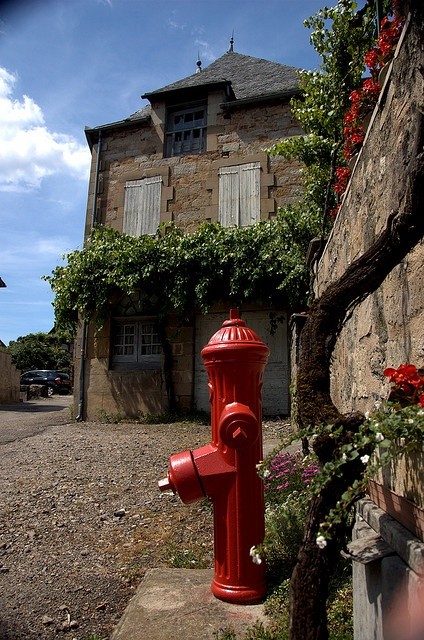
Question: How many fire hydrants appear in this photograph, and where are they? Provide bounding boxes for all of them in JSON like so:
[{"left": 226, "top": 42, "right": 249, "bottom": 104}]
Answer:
[{"left": 157, "top": 310, "right": 269, "bottom": 604}]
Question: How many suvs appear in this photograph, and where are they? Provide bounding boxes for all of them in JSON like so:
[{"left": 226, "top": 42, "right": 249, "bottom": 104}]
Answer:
[{"left": 20, "top": 369, "right": 71, "bottom": 396}]
